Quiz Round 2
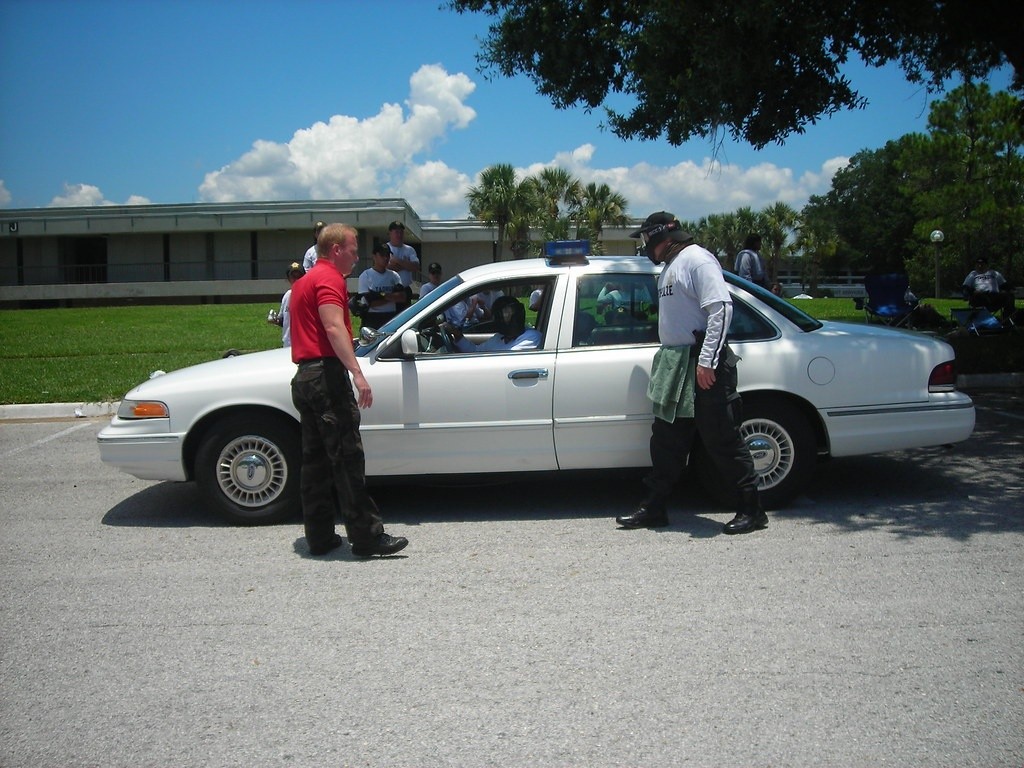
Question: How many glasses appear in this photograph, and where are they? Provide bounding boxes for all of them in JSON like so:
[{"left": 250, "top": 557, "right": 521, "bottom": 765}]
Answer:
[
  {"left": 638, "top": 221, "right": 676, "bottom": 248},
  {"left": 976, "top": 261, "right": 986, "bottom": 266}
]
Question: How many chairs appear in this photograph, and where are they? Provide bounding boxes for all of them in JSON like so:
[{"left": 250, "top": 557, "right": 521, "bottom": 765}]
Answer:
[
  {"left": 573, "top": 310, "right": 596, "bottom": 347},
  {"left": 948, "top": 306, "right": 1007, "bottom": 339},
  {"left": 861, "top": 270, "right": 912, "bottom": 329},
  {"left": 968, "top": 287, "right": 1005, "bottom": 329}
]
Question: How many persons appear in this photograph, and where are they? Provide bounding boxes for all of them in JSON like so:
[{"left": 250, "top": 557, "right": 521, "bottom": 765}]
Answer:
[
  {"left": 962, "top": 257, "right": 1015, "bottom": 322},
  {"left": 770, "top": 282, "right": 783, "bottom": 296},
  {"left": 616, "top": 211, "right": 769, "bottom": 534},
  {"left": 419, "top": 263, "right": 443, "bottom": 299},
  {"left": 734, "top": 233, "right": 766, "bottom": 287},
  {"left": 290, "top": 223, "right": 409, "bottom": 557},
  {"left": 357, "top": 221, "right": 418, "bottom": 330},
  {"left": 268, "top": 221, "right": 327, "bottom": 347},
  {"left": 597, "top": 282, "right": 645, "bottom": 314},
  {"left": 444, "top": 284, "right": 546, "bottom": 351}
]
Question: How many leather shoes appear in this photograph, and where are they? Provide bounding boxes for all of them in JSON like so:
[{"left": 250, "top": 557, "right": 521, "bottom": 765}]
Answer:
[
  {"left": 352, "top": 534, "right": 409, "bottom": 557},
  {"left": 723, "top": 509, "right": 770, "bottom": 535},
  {"left": 616, "top": 506, "right": 669, "bottom": 528},
  {"left": 307, "top": 533, "right": 344, "bottom": 557}
]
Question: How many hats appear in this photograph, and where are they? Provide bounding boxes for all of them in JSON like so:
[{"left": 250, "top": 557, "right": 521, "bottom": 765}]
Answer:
[
  {"left": 285, "top": 262, "right": 306, "bottom": 276},
  {"left": 389, "top": 221, "right": 405, "bottom": 231},
  {"left": 628, "top": 211, "right": 681, "bottom": 238},
  {"left": 314, "top": 221, "right": 328, "bottom": 232},
  {"left": 429, "top": 262, "right": 442, "bottom": 273},
  {"left": 374, "top": 244, "right": 394, "bottom": 257}
]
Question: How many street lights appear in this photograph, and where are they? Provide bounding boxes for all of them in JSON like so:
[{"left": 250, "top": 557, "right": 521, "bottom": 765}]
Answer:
[{"left": 931, "top": 230, "right": 945, "bottom": 298}]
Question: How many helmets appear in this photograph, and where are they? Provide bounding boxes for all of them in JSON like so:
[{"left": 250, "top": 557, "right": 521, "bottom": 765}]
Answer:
[{"left": 491, "top": 296, "right": 527, "bottom": 334}]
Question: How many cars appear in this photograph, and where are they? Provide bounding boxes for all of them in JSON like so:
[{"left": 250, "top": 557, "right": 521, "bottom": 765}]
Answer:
[{"left": 96, "top": 239, "right": 976, "bottom": 527}]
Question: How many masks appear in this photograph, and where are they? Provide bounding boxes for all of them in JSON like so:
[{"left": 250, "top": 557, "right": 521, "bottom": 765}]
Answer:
[{"left": 644, "top": 233, "right": 670, "bottom": 266}]
